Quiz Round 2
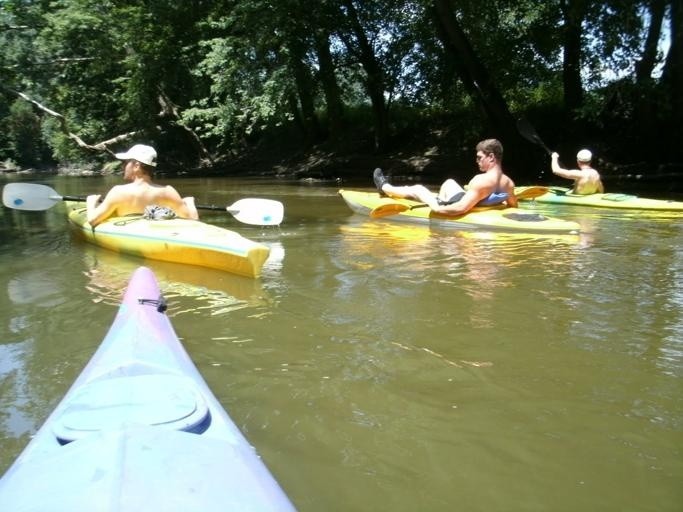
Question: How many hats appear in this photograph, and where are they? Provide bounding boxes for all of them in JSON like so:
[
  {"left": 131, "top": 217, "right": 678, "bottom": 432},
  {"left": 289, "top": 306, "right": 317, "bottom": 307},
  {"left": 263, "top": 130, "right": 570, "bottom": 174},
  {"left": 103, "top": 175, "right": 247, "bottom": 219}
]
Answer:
[
  {"left": 114, "top": 144, "right": 157, "bottom": 167},
  {"left": 577, "top": 149, "right": 592, "bottom": 162}
]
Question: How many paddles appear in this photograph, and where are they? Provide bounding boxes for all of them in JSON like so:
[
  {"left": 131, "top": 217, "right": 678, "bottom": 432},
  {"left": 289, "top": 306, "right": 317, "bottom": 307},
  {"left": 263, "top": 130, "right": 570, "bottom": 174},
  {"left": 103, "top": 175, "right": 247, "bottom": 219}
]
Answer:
[
  {"left": 517, "top": 117, "right": 568, "bottom": 170},
  {"left": 2, "top": 183, "right": 284, "bottom": 225},
  {"left": 369, "top": 187, "right": 548, "bottom": 220}
]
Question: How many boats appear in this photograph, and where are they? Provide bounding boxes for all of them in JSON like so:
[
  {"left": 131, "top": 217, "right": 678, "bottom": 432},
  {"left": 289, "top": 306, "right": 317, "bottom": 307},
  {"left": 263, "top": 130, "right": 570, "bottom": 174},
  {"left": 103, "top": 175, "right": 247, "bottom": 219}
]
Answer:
[
  {"left": 0, "top": 266, "right": 298, "bottom": 512},
  {"left": 467, "top": 185, "right": 682, "bottom": 219},
  {"left": 64, "top": 199, "right": 269, "bottom": 278},
  {"left": 338, "top": 190, "right": 582, "bottom": 236}
]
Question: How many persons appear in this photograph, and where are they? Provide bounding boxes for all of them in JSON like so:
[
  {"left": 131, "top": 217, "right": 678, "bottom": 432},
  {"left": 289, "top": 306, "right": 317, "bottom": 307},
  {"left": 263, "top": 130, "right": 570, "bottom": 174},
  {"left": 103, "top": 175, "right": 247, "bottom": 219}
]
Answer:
[
  {"left": 551, "top": 148, "right": 605, "bottom": 196},
  {"left": 373, "top": 137, "right": 519, "bottom": 216},
  {"left": 87, "top": 143, "right": 201, "bottom": 229}
]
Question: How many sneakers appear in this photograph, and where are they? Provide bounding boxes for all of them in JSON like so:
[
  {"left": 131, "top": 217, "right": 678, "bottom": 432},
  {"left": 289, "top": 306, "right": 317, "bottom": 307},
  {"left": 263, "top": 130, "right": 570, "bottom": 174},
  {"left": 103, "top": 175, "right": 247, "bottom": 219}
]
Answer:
[{"left": 374, "top": 168, "right": 387, "bottom": 196}]
